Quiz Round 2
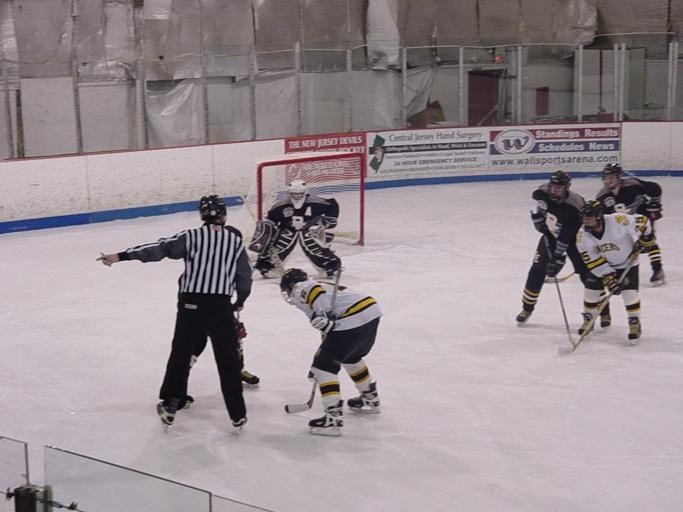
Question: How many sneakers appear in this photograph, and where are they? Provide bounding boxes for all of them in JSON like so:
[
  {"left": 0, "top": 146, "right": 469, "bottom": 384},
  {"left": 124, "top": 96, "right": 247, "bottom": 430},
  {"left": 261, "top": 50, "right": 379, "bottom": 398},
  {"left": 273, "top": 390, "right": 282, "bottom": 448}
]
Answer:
[
  {"left": 157, "top": 402, "right": 179, "bottom": 426},
  {"left": 240, "top": 369, "right": 259, "bottom": 384},
  {"left": 578, "top": 312, "right": 592, "bottom": 336},
  {"left": 347, "top": 391, "right": 381, "bottom": 407},
  {"left": 600, "top": 314, "right": 611, "bottom": 328},
  {"left": 650, "top": 269, "right": 664, "bottom": 283},
  {"left": 308, "top": 406, "right": 344, "bottom": 429},
  {"left": 232, "top": 416, "right": 248, "bottom": 430},
  {"left": 516, "top": 309, "right": 532, "bottom": 322},
  {"left": 627, "top": 317, "right": 641, "bottom": 339}
]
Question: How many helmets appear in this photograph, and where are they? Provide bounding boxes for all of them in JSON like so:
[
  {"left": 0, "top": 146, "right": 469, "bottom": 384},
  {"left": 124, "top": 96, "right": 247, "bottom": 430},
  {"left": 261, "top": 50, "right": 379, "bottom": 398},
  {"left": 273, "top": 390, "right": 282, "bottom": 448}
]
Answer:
[
  {"left": 579, "top": 199, "right": 605, "bottom": 232},
  {"left": 548, "top": 170, "right": 571, "bottom": 202},
  {"left": 278, "top": 268, "right": 307, "bottom": 305},
  {"left": 199, "top": 194, "right": 226, "bottom": 220},
  {"left": 600, "top": 163, "right": 622, "bottom": 191},
  {"left": 288, "top": 179, "right": 308, "bottom": 210}
]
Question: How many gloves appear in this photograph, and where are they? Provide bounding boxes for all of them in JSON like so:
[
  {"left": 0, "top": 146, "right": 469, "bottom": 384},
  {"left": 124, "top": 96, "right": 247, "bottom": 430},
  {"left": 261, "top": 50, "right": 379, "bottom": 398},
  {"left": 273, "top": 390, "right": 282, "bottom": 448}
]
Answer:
[
  {"left": 546, "top": 254, "right": 565, "bottom": 277},
  {"left": 95, "top": 252, "right": 119, "bottom": 267},
  {"left": 603, "top": 271, "right": 621, "bottom": 296},
  {"left": 309, "top": 313, "right": 339, "bottom": 335},
  {"left": 635, "top": 234, "right": 656, "bottom": 253},
  {"left": 530, "top": 210, "right": 546, "bottom": 233},
  {"left": 645, "top": 204, "right": 662, "bottom": 222}
]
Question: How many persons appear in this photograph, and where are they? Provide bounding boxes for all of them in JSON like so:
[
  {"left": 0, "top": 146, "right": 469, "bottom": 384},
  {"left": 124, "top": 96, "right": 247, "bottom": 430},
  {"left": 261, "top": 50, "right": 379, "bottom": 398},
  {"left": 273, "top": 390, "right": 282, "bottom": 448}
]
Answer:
[
  {"left": 279, "top": 267, "right": 383, "bottom": 430},
  {"left": 248, "top": 179, "right": 341, "bottom": 277},
  {"left": 575, "top": 199, "right": 654, "bottom": 341},
  {"left": 514, "top": 170, "right": 611, "bottom": 328},
  {"left": 95, "top": 193, "right": 253, "bottom": 428},
  {"left": 185, "top": 224, "right": 261, "bottom": 404},
  {"left": 595, "top": 163, "right": 666, "bottom": 283}
]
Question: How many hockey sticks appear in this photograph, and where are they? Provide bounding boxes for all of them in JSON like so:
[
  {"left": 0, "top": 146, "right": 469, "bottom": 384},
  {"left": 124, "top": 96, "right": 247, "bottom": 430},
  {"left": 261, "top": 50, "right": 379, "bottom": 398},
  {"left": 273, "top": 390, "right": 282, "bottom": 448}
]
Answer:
[
  {"left": 543, "top": 236, "right": 577, "bottom": 345},
  {"left": 285, "top": 267, "right": 343, "bottom": 414},
  {"left": 558, "top": 264, "right": 632, "bottom": 355},
  {"left": 546, "top": 271, "right": 575, "bottom": 283}
]
